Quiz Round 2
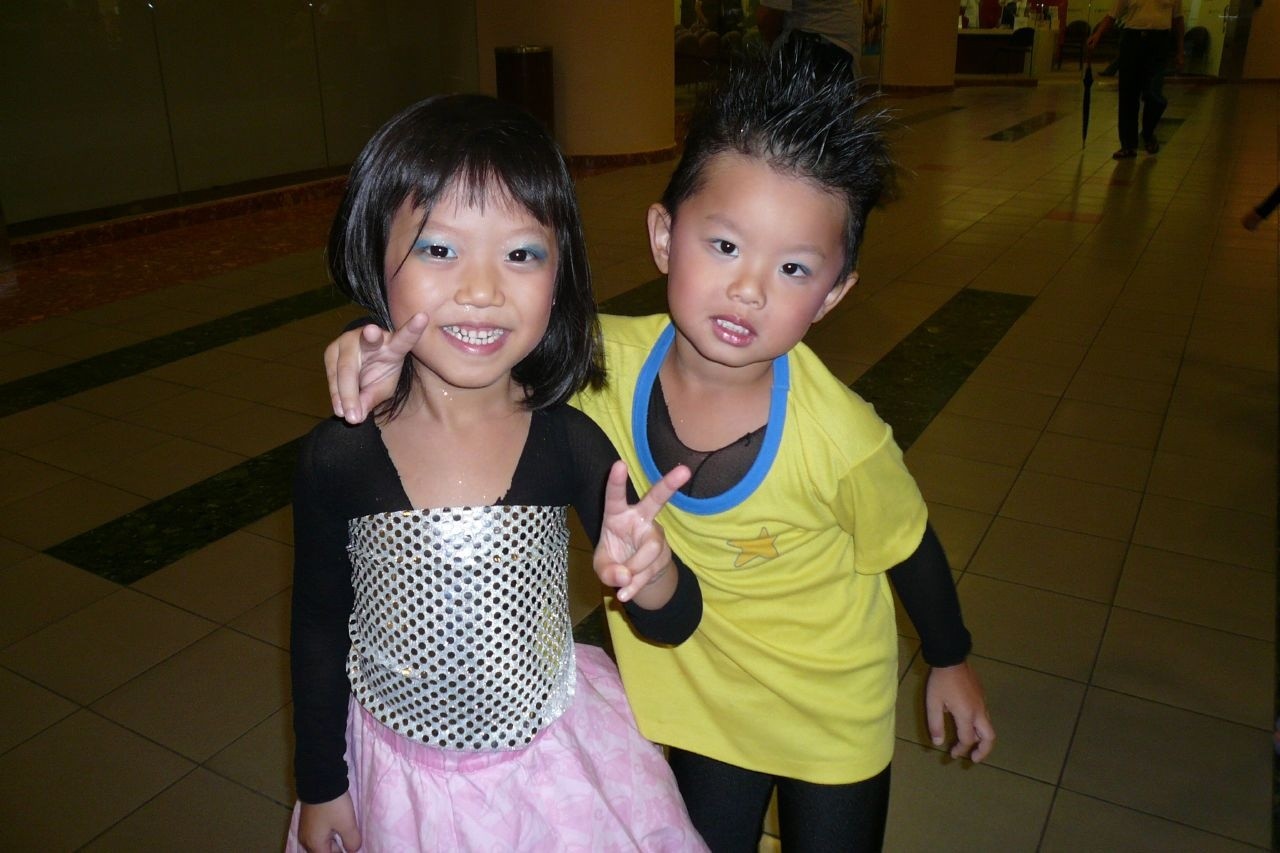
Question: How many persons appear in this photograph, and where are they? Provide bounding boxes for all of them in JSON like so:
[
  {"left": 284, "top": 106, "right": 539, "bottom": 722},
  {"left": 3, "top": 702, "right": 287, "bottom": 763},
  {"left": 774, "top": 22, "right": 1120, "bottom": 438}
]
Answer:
[
  {"left": 323, "top": 42, "right": 997, "bottom": 853},
  {"left": 285, "top": 91, "right": 712, "bottom": 853},
  {"left": 1242, "top": 184, "right": 1280, "bottom": 231},
  {"left": 1087, "top": 0, "right": 1185, "bottom": 158},
  {"left": 694, "top": 0, "right": 865, "bottom": 90}
]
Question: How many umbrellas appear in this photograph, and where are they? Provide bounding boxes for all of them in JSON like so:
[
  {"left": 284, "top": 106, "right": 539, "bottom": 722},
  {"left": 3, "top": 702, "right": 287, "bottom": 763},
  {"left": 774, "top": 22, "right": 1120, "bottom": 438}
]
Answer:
[{"left": 1082, "top": 43, "right": 1093, "bottom": 151}]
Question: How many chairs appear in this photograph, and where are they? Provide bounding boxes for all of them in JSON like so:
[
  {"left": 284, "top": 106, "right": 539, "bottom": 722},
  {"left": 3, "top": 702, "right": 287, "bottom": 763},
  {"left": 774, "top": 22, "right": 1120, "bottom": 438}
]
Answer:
[
  {"left": 1056, "top": 20, "right": 1091, "bottom": 72},
  {"left": 1090, "top": 20, "right": 1121, "bottom": 64},
  {"left": 674, "top": 20, "right": 763, "bottom": 96},
  {"left": 1184, "top": 26, "right": 1211, "bottom": 76},
  {"left": 994, "top": 27, "right": 1036, "bottom": 78}
]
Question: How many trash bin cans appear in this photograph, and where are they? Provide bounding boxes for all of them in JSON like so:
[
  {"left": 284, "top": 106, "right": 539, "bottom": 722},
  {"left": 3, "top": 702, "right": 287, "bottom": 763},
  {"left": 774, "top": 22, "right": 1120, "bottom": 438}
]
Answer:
[{"left": 494, "top": 44, "right": 556, "bottom": 141}]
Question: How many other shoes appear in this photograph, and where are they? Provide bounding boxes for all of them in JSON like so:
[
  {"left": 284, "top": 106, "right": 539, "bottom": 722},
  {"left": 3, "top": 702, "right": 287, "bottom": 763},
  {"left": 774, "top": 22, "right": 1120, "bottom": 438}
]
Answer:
[
  {"left": 1113, "top": 149, "right": 1137, "bottom": 159},
  {"left": 1138, "top": 128, "right": 1159, "bottom": 155}
]
